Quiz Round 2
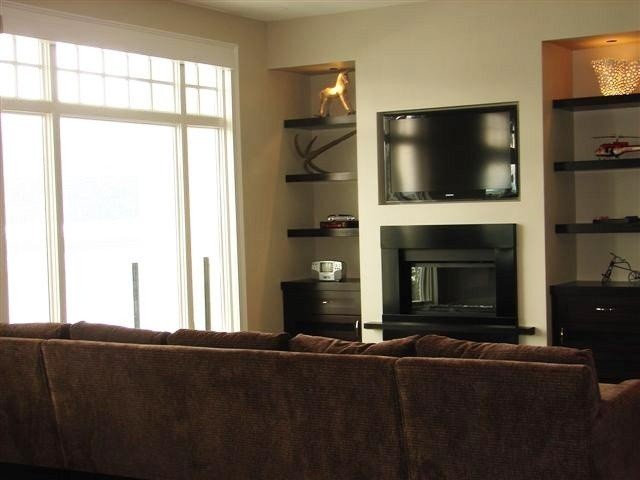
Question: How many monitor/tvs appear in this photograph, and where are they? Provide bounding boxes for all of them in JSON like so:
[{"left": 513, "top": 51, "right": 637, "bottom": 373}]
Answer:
[{"left": 383, "top": 103, "right": 517, "bottom": 202}]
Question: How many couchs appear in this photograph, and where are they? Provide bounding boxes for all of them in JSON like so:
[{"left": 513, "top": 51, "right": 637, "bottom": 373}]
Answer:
[{"left": 1, "top": 321, "right": 640, "bottom": 480}]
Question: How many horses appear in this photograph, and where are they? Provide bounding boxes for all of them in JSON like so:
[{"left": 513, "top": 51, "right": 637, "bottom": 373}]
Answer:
[{"left": 318, "top": 71, "right": 355, "bottom": 117}]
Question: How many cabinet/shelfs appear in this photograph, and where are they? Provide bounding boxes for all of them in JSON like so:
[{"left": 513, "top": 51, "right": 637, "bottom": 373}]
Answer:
[
  {"left": 282, "top": 114, "right": 360, "bottom": 343},
  {"left": 553, "top": 92, "right": 640, "bottom": 382}
]
